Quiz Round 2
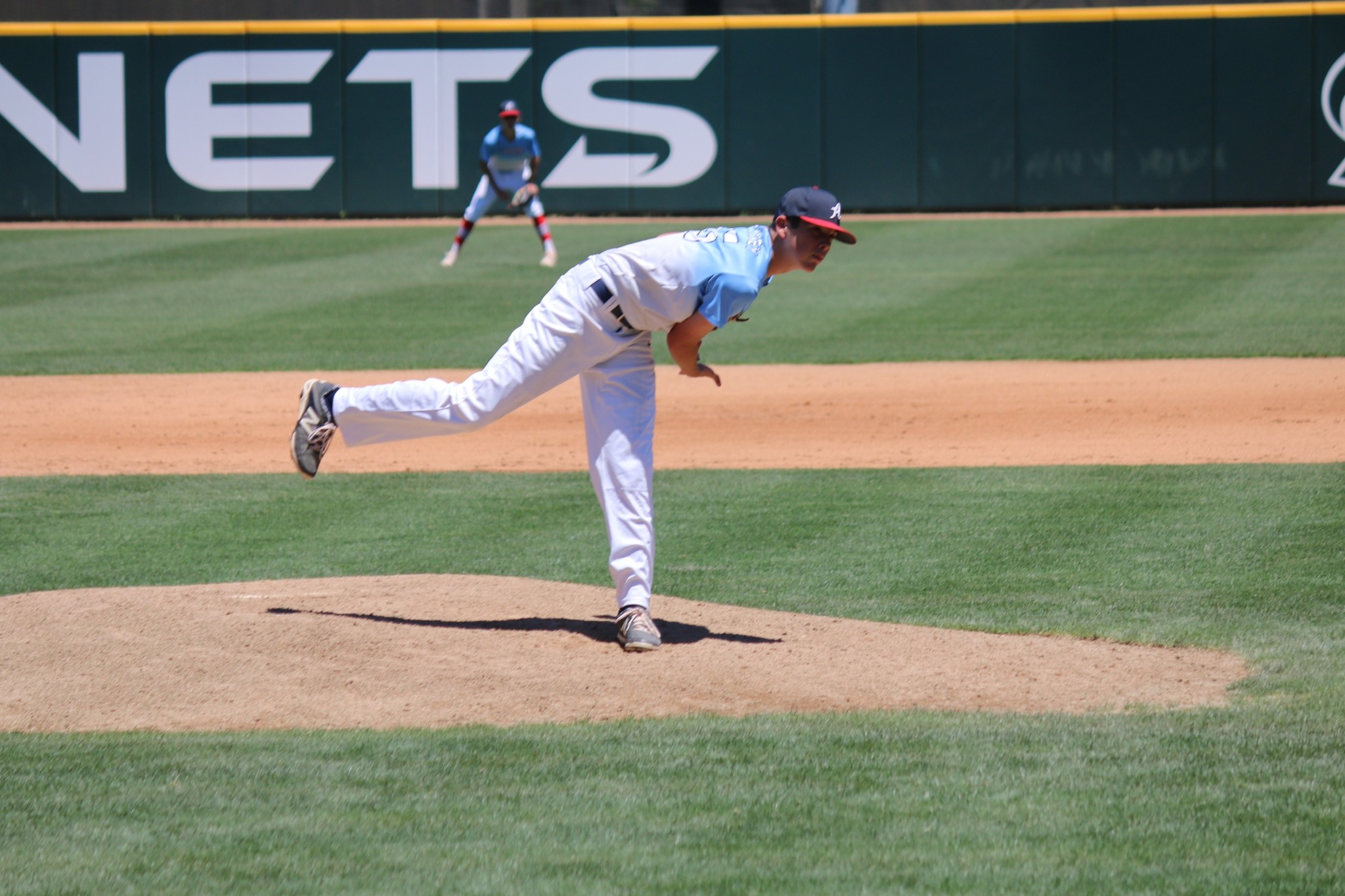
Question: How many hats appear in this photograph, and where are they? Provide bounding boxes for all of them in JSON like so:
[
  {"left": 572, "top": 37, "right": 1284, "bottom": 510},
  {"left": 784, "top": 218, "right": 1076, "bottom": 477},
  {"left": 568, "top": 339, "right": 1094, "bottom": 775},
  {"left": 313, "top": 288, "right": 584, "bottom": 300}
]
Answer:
[
  {"left": 775, "top": 184, "right": 858, "bottom": 246},
  {"left": 495, "top": 98, "right": 522, "bottom": 117}
]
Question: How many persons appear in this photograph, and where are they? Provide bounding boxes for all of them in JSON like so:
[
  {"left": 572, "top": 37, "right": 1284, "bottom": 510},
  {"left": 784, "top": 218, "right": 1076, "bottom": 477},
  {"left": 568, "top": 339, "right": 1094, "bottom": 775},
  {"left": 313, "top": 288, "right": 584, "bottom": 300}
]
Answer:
[
  {"left": 290, "top": 186, "right": 856, "bottom": 652},
  {"left": 437, "top": 101, "right": 558, "bottom": 268}
]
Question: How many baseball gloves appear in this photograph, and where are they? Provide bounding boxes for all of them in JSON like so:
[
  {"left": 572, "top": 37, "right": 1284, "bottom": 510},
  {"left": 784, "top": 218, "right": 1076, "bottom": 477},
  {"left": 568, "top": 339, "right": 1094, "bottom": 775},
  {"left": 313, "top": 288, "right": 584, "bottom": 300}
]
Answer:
[{"left": 508, "top": 184, "right": 540, "bottom": 208}]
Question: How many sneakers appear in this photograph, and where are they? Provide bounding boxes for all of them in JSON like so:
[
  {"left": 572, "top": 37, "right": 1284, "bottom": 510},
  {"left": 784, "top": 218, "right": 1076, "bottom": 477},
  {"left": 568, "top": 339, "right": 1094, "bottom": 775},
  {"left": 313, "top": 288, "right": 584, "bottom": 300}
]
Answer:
[
  {"left": 614, "top": 606, "right": 663, "bottom": 653},
  {"left": 539, "top": 249, "right": 558, "bottom": 268},
  {"left": 289, "top": 376, "right": 339, "bottom": 480},
  {"left": 441, "top": 250, "right": 460, "bottom": 268}
]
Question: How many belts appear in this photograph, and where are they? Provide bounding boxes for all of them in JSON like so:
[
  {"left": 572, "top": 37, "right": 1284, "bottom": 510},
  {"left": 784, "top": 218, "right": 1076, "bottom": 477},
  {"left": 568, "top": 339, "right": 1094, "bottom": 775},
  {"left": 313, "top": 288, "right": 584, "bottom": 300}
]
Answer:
[{"left": 579, "top": 257, "right": 634, "bottom": 333}]
